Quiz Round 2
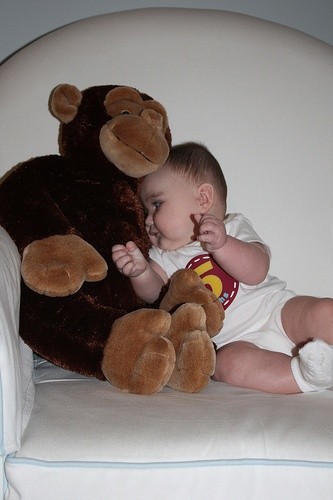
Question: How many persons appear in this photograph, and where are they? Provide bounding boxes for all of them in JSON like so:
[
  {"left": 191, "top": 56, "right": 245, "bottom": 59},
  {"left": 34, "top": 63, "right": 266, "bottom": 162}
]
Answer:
[{"left": 111, "top": 140, "right": 332, "bottom": 395}]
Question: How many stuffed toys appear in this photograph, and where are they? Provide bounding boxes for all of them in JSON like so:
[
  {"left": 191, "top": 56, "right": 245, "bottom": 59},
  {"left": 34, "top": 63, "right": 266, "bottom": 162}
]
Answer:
[{"left": 1, "top": 79, "right": 225, "bottom": 399}]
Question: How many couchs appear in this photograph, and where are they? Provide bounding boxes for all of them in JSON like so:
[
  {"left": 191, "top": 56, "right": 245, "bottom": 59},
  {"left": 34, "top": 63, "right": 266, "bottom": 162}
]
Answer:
[{"left": 0, "top": 7, "right": 333, "bottom": 499}]
